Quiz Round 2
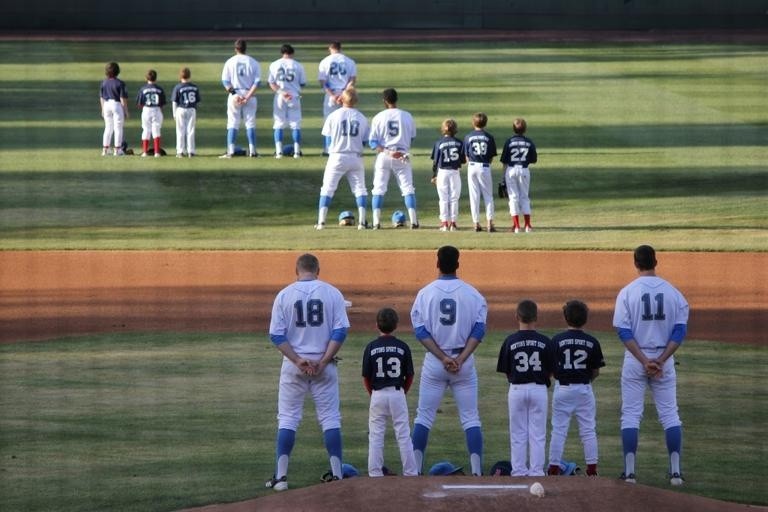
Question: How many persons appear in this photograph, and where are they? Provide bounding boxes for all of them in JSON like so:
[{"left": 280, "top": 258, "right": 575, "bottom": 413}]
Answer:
[
  {"left": 360, "top": 306, "right": 419, "bottom": 477},
  {"left": 316, "top": 84, "right": 535, "bottom": 236},
  {"left": 265, "top": 256, "right": 350, "bottom": 490},
  {"left": 610, "top": 244, "right": 690, "bottom": 483},
  {"left": 98, "top": 62, "right": 201, "bottom": 160},
  {"left": 494, "top": 300, "right": 559, "bottom": 476},
  {"left": 548, "top": 300, "right": 605, "bottom": 475},
  {"left": 221, "top": 35, "right": 358, "bottom": 156},
  {"left": 410, "top": 245, "right": 488, "bottom": 475}
]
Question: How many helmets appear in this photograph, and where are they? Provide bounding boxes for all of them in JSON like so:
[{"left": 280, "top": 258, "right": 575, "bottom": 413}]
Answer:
[
  {"left": 339, "top": 211, "right": 357, "bottom": 226},
  {"left": 391, "top": 210, "right": 407, "bottom": 228},
  {"left": 341, "top": 463, "right": 359, "bottom": 479},
  {"left": 428, "top": 462, "right": 463, "bottom": 475}
]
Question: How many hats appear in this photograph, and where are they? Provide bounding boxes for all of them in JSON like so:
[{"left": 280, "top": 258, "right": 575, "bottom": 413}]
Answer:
[{"left": 560, "top": 461, "right": 576, "bottom": 477}]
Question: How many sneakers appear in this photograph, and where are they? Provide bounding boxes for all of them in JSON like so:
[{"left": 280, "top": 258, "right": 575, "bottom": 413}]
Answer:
[
  {"left": 273, "top": 155, "right": 283, "bottom": 159},
  {"left": 440, "top": 223, "right": 533, "bottom": 232},
  {"left": 102, "top": 147, "right": 193, "bottom": 158},
  {"left": 265, "top": 474, "right": 288, "bottom": 492},
  {"left": 411, "top": 221, "right": 420, "bottom": 229},
  {"left": 670, "top": 471, "right": 685, "bottom": 486},
  {"left": 293, "top": 154, "right": 301, "bottom": 159},
  {"left": 618, "top": 471, "right": 636, "bottom": 483},
  {"left": 218, "top": 154, "right": 233, "bottom": 159},
  {"left": 250, "top": 153, "right": 262, "bottom": 157},
  {"left": 358, "top": 221, "right": 381, "bottom": 229},
  {"left": 315, "top": 222, "right": 326, "bottom": 230}
]
